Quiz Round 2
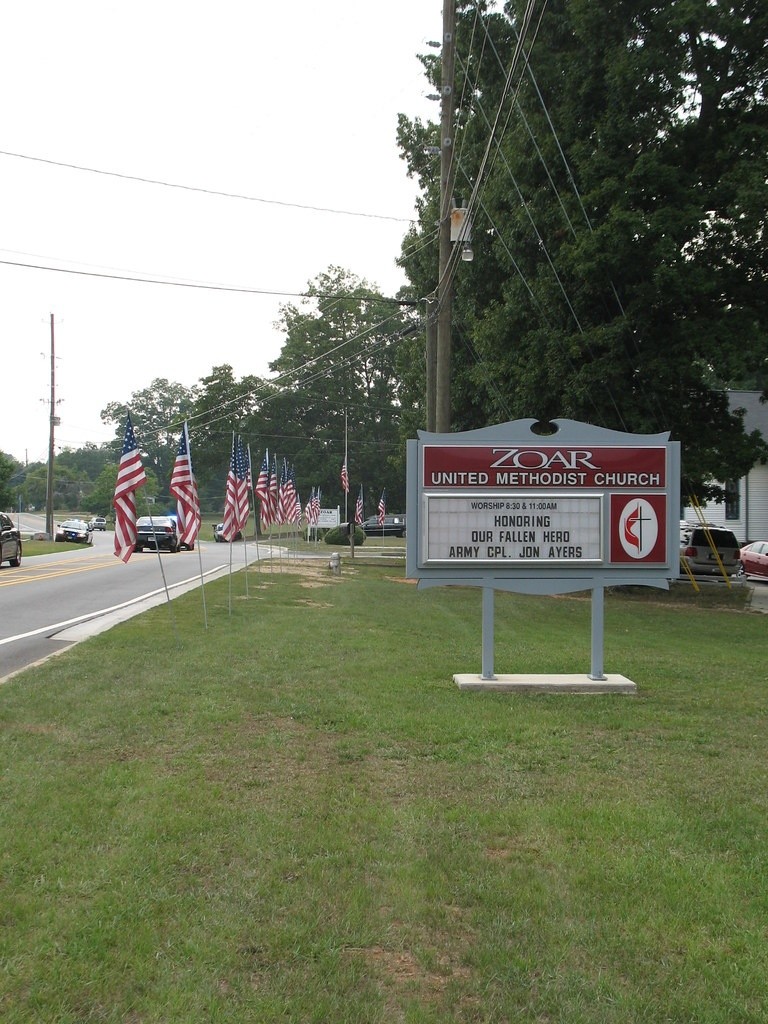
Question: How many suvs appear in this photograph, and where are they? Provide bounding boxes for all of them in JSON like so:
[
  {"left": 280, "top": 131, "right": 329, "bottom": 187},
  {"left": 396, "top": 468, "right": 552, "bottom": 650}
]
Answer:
[
  {"left": 680, "top": 524, "right": 741, "bottom": 576},
  {"left": 358, "top": 515, "right": 406, "bottom": 538},
  {"left": 89, "top": 517, "right": 107, "bottom": 531}
]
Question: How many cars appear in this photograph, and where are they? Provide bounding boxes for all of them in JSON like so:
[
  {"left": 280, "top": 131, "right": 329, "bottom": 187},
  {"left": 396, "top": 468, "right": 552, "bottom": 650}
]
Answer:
[
  {"left": 0, "top": 513, "right": 22, "bottom": 567},
  {"left": 736, "top": 541, "right": 768, "bottom": 579},
  {"left": 214, "top": 523, "right": 241, "bottom": 542},
  {"left": 135, "top": 516, "right": 181, "bottom": 553},
  {"left": 55, "top": 520, "right": 93, "bottom": 544}
]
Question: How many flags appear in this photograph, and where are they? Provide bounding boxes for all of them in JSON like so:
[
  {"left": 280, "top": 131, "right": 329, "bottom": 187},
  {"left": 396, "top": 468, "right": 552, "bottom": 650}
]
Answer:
[
  {"left": 169, "top": 421, "right": 202, "bottom": 549},
  {"left": 235, "top": 440, "right": 250, "bottom": 530},
  {"left": 270, "top": 458, "right": 321, "bottom": 530},
  {"left": 220, "top": 436, "right": 241, "bottom": 542},
  {"left": 112, "top": 414, "right": 148, "bottom": 565},
  {"left": 245, "top": 443, "right": 253, "bottom": 491},
  {"left": 378, "top": 490, "right": 386, "bottom": 525},
  {"left": 255, "top": 454, "right": 272, "bottom": 532},
  {"left": 341, "top": 458, "right": 350, "bottom": 494},
  {"left": 354, "top": 495, "right": 363, "bottom": 524}
]
